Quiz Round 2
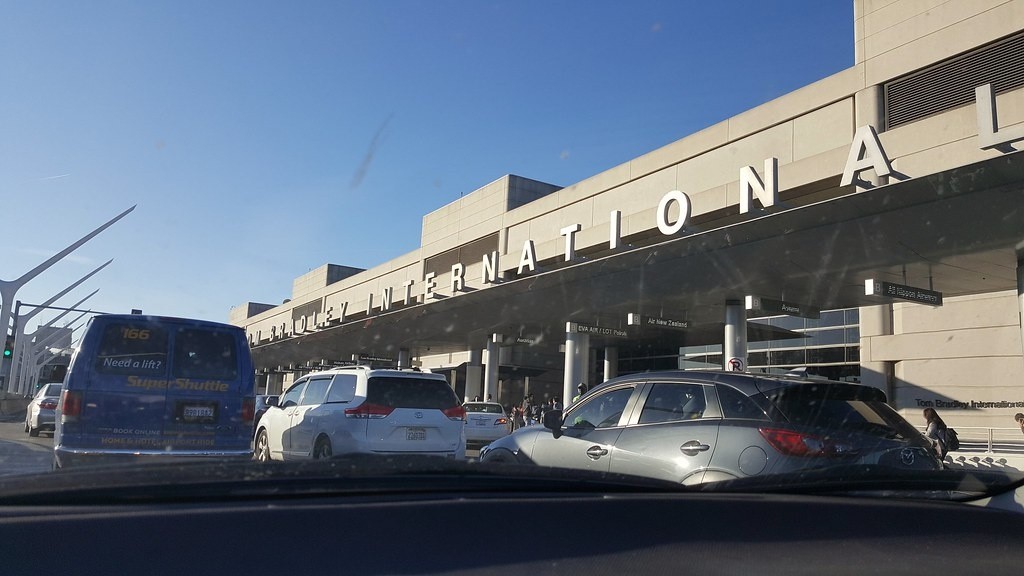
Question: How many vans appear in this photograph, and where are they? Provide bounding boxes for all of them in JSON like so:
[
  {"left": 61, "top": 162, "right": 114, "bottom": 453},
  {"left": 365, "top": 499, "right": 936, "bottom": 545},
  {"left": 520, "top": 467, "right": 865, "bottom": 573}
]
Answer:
[{"left": 51, "top": 308, "right": 255, "bottom": 470}]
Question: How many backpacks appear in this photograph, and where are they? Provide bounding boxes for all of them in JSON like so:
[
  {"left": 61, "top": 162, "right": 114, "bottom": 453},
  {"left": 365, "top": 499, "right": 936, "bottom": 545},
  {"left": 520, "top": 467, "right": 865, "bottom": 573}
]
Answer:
[{"left": 928, "top": 422, "right": 960, "bottom": 451}]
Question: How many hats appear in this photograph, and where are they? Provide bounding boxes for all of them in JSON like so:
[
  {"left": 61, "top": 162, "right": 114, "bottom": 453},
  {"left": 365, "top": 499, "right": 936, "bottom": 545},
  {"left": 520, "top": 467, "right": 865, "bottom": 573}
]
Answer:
[{"left": 578, "top": 383, "right": 587, "bottom": 390}]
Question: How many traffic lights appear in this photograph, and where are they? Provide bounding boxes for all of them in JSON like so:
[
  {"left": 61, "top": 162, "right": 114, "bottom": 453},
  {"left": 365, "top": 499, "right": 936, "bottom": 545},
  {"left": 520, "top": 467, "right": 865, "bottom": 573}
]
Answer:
[{"left": 3, "top": 335, "right": 16, "bottom": 358}]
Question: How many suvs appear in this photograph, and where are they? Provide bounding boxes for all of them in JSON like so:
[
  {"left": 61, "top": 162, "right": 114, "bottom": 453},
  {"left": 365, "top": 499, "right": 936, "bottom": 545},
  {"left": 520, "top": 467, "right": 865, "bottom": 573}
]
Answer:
[
  {"left": 474, "top": 367, "right": 943, "bottom": 487},
  {"left": 252, "top": 364, "right": 467, "bottom": 462}
]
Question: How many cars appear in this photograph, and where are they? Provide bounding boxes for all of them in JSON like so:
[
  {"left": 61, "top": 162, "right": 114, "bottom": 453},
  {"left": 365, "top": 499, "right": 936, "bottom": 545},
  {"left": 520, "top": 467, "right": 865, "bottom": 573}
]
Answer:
[
  {"left": 24, "top": 383, "right": 63, "bottom": 436},
  {"left": 461, "top": 401, "right": 513, "bottom": 444},
  {"left": 255, "top": 394, "right": 279, "bottom": 427}
]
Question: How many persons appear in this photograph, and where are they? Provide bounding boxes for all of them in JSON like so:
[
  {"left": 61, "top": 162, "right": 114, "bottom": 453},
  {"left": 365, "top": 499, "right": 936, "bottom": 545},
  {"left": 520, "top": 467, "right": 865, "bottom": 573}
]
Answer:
[
  {"left": 553, "top": 396, "right": 562, "bottom": 409},
  {"left": 1015, "top": 413, "right": 1024, "bottom": 433},
  {"left": 523, "top": 393, "right": 533, "bottom": 426},
  {"left": 470, "top": 396, "right": 483, "bottom": 411},
  {"left": 531, "top": 398, "right": 553, "bottom": 425},
  {"left": 573, "top": 383, "right": 587, "bottom": 402},
  {"left": 924, "top": 408, "right": 948, "bottom": 461}
]
